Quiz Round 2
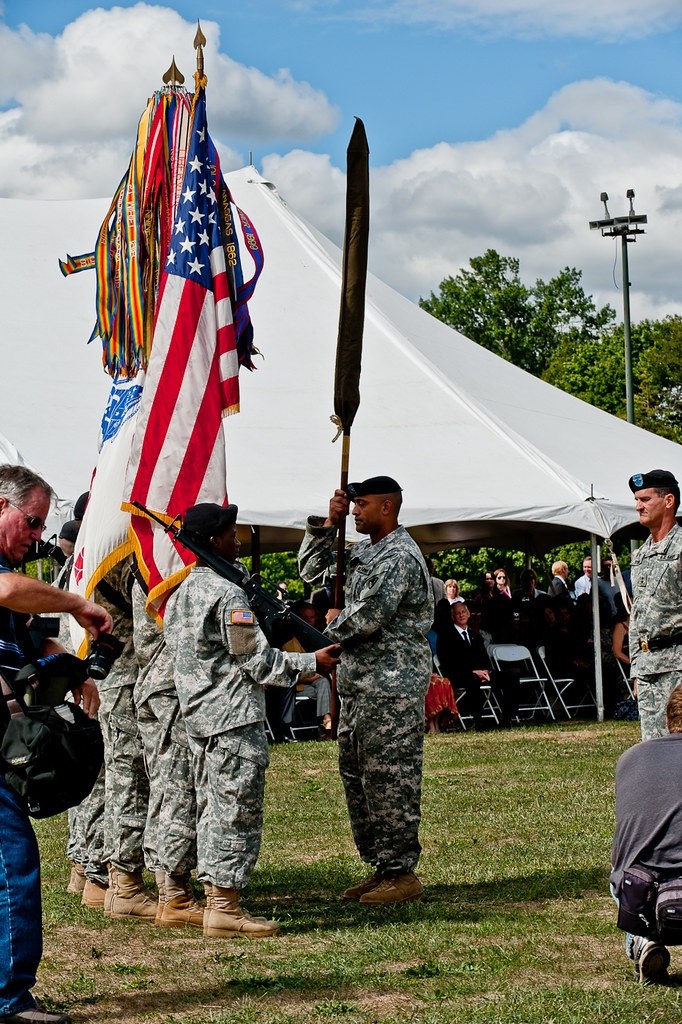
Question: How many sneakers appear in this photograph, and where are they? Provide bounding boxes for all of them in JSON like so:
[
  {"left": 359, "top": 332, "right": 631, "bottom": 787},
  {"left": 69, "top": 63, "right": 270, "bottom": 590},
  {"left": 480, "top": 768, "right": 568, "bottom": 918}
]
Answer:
[
  {"left": 358, "top": 872, "right": 424, "bottom": 905},
  {"left": 342, "top": 872, "right": 384, "bottom": 899}
]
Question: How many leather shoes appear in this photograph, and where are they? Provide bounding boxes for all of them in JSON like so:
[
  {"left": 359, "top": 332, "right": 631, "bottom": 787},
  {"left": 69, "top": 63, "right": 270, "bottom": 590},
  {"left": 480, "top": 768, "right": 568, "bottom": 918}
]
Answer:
[{"left": 277, "top": 734, "right": 301, "bottom": 745}]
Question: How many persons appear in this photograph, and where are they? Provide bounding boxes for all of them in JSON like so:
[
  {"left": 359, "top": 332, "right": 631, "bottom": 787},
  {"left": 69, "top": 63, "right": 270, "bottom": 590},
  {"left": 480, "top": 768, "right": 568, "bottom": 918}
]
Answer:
[
  {"left": 629, "top": 470, "right": 682, "bottom": 742},
  {"left": 0, "top": 464, "right": 112, "bottom": 1024},
  {"left": 57, "top": 494, "right": 638, "bottom": 936},
  {"left": 296, "top": 475, "right": 433, "bottom": 901},
  {"left": 610, "top": 683, "right": 682, "bottom": 982}
]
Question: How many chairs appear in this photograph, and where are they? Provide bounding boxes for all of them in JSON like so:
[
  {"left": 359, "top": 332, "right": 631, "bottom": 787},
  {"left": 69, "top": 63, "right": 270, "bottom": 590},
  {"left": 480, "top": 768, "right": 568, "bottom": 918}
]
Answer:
[{"left": 264, "top": 643, "right": 636, "bottom": 742}]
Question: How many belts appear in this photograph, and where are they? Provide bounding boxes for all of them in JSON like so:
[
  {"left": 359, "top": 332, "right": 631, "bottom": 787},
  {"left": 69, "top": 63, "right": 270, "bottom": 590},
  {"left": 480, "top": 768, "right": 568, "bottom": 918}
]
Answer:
[{"left": 639, "top": 636, "right": 682, "bottom": 652}]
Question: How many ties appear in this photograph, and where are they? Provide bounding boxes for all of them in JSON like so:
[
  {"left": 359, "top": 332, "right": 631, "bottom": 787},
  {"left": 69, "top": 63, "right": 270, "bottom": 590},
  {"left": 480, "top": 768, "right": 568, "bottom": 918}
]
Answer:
[{"left": 462, "top": 631, "right": 470, "bottom": 646}]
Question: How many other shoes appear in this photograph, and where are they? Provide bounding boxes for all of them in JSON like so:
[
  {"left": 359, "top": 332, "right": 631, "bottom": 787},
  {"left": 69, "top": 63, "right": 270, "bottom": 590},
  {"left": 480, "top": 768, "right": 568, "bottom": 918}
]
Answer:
[
  {"left": 0, "top": 1010, "right": 70, "bottom": 1024},
  {"left": 631, "top": 936, "right": 670, "bottom": 986}
]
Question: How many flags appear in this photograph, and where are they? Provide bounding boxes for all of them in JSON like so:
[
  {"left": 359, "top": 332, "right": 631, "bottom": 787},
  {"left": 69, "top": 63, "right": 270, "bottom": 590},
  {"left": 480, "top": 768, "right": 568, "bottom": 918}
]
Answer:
[{"left": 67, "top": 75, "right": 239, "bottom": 659}]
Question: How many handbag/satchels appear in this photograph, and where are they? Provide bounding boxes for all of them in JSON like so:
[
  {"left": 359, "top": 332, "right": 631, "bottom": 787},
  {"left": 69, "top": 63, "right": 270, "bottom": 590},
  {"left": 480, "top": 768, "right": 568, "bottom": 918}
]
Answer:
[
  {"left": 616, "top": 865, "right": 682, "bottom": 946},
  {"left": 0, "top": 701, "right": 104, "bottom": 819}
]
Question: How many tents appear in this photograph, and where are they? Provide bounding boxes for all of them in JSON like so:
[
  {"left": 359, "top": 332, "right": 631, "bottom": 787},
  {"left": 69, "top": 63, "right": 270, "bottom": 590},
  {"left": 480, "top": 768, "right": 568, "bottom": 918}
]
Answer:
[{"left": 0, "top": 167, "right": 682, "bottom": 723}]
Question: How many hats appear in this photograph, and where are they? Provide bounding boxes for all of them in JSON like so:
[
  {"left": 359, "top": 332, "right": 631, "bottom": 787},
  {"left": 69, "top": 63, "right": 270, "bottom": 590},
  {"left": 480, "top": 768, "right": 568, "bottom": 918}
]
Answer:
[
  {"left": 183, "top": 503, "right": 239, "bottom": 549},
  {"left": 344, "top": 476, "right": 403, "bottom": 503},
  {"left": 629, "top": 470, "right": 678, "bottom": 493}
]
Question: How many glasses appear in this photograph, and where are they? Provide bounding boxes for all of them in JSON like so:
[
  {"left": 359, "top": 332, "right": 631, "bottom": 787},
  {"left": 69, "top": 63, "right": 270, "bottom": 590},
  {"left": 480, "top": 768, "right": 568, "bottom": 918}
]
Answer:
[
  {"left": 497, "top": 576, "right": 506, "bottom": 580},
  {"left": 8, "top": 501, "right": 47, "bottom": 533},
  {"left": 521, "top": 574, "right": 535, "bottom": 581}
]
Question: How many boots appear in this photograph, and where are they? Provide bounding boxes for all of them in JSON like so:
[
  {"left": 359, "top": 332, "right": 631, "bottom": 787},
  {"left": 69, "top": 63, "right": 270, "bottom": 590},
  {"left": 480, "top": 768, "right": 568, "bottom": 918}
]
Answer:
[
  {"left": 104, "top": 870, "right": 158, "bottom": 920},
  {"left": 80, "top": 880, "right": 107, "bottom": 909},
  {"left": 155, "top": 882, "right": 207, "bottom": 927},
  {"left": 203, "top": 883, "right": 280, "bottom": 938},
  {"left": 66, "top": 863, "right": 87, "bottom": 894}
]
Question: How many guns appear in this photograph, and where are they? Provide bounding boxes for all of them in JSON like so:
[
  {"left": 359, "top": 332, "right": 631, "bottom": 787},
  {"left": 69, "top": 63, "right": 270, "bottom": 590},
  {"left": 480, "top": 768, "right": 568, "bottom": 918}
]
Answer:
[{"left": 130, "top": 499, "right": 345, "bottom": 659}]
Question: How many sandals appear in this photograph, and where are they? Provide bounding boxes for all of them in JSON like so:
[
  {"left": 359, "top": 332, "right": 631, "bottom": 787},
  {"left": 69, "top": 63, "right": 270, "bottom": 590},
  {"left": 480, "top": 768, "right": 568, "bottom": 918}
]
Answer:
[{"left": 319, "top": 718, "right": 332, "bottom": 730}]
